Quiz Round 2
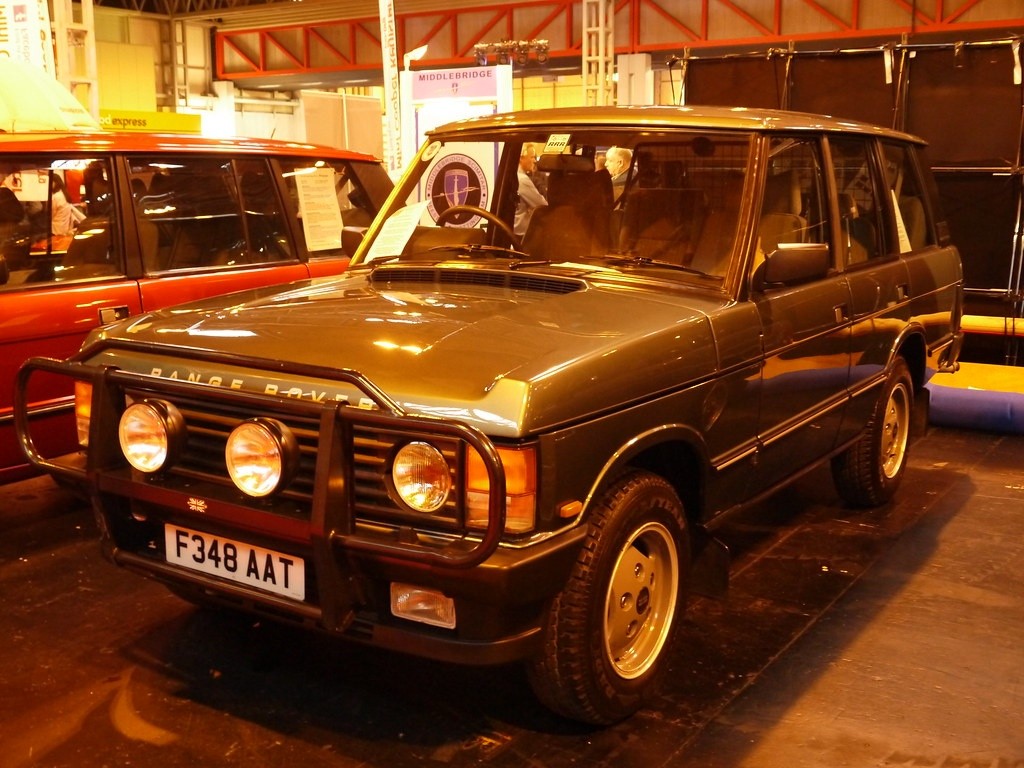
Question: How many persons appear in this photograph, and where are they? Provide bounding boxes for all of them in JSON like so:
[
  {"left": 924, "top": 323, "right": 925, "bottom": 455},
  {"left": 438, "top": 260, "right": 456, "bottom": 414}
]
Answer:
[
  {"left": 513, "top": 142, "right": 548, "bottom": 244},
  {"left": 51, "top": 174, "right": 71, "bottom": 235},
  {"left": 605, "top": 145, "right": 638, "bottom": 210}
]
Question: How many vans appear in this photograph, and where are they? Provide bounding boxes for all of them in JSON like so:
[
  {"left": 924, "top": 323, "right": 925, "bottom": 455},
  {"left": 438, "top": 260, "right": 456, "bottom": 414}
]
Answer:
[{"left": 1, "top": 130, "right": 408, "bottom": 490}]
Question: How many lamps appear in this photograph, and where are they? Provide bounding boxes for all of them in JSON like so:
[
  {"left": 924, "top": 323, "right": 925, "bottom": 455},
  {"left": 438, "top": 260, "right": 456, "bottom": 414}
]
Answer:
[{"left": 474, "top": 39, "right": 549, "bottom": 66}]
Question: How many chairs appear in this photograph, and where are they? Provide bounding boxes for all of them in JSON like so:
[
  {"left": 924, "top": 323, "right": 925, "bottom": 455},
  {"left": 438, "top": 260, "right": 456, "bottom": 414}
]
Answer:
[
  {"left": 837, "top": 192, "right": 868, "bottom": 266},
  {"left": 688, "top": 171, "right": 806, "bottom": 284},
  {"left": 891, "top": 193, "right": 929, "bottom": 253},
  {"left": 131, "top": 171, "right": 290, "bottom": 269},
  {"left": 616, "top": 161, "right": 708, "bottom": 267},
  {"left": 63, "top": 176, "right": 119, "bottom": 265},
  {"left": 0, "top": 190, "right": 30, "bottom": 269},
  {"left": 518, "top": 168, "right": 616, "bottom": 262}
]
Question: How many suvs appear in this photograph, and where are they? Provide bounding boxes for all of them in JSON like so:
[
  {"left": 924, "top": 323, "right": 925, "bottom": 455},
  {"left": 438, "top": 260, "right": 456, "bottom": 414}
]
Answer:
[{"left": 12, "top": 104, "right": 967, "bottom": 731}]
